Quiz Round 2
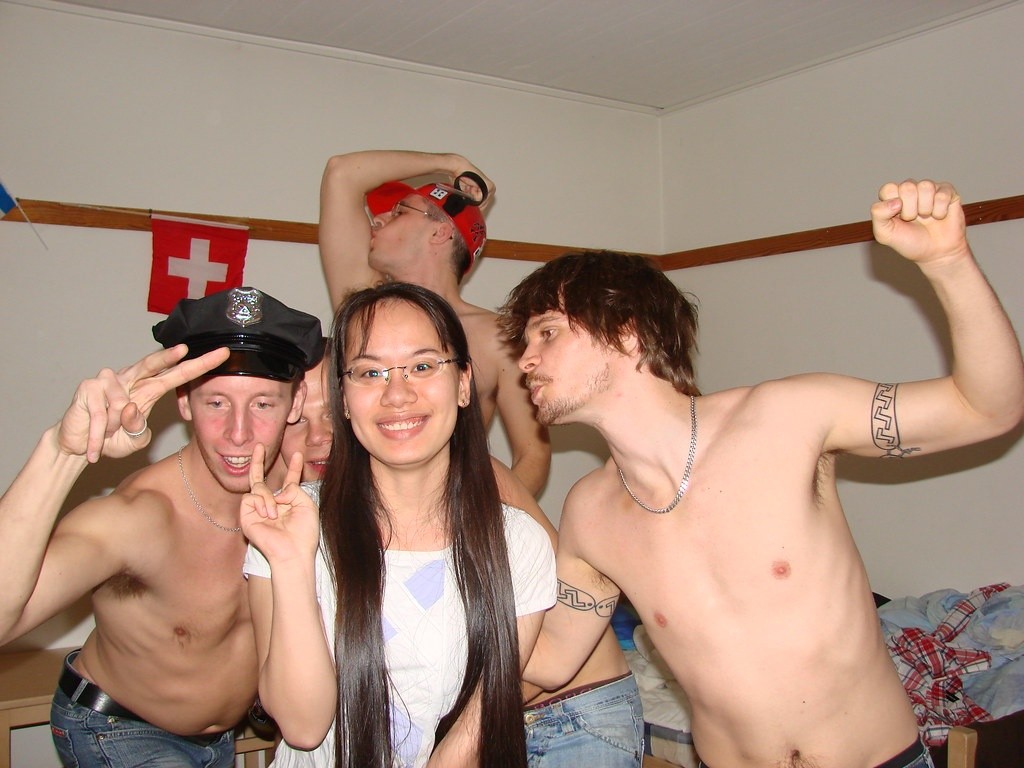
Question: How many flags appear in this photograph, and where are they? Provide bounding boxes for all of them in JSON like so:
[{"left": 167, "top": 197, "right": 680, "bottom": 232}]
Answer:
[{"left": 146, "top": 212, "right": 249, "bottom": 315}]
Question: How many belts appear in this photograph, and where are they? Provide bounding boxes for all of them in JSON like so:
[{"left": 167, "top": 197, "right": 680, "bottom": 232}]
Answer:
[
  {"left": 59, "top": 650, "right": 223, "bottom": 744},
  {"left": 699, "top": 736, "right": 924, "bottom": 768}
]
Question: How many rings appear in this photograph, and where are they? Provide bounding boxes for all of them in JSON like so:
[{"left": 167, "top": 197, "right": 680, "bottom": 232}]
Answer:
[{"left": 123, "top": 417, "right": 148, "bottom": 439}]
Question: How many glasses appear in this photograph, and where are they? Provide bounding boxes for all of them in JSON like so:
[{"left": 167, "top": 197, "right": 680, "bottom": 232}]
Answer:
[
  {"left": 392, "top": 201, "right": 456, "bottom": 241},
  {"left": 337, "top": 353, "right": 464, "bottom": 383}
]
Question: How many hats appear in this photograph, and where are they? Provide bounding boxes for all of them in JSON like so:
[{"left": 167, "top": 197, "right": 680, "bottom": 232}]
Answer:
[
  {"left": 152, "top": 286, "right": 327, "bottom": 382},
  {"left": 366, "top": 180, "right": 486, "bottom": 275}
]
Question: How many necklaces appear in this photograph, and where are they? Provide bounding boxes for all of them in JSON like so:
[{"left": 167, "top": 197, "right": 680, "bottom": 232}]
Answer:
[
  {"left": 617, "top": 395, "right": 697, "bottom": 514},
  {"left": 179, "top": 446, "right": 266, "bottom": 531}
]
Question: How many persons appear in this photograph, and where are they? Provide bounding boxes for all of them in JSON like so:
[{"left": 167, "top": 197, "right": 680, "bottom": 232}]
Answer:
[
  {"left": 498, "top": 179, "right": 1024, "bottom": 768},
  {"left": 0, "top": 149, "right": 645, "bottom": 768}
]
